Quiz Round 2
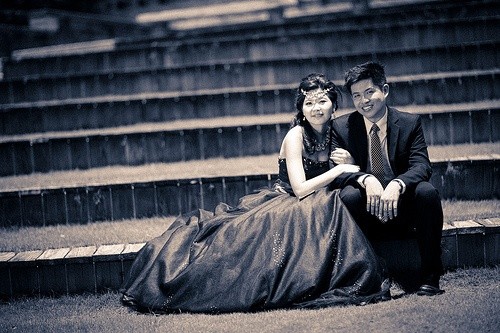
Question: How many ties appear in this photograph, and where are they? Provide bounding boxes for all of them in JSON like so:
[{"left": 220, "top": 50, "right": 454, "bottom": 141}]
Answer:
[{"left": 371, "top": 124, "right": 387, "bottom": 190}]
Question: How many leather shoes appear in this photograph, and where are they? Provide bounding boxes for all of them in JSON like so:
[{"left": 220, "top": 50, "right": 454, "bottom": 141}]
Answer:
[
  {"left": 418, "top": 283, "right": 440, "bottom": 296},
  {"left": 381, "top": 290, "right": 392, "bottom": 301}
]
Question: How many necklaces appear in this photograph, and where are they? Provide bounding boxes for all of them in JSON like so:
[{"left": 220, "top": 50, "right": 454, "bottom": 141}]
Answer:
[{"left": 309, "top": 122, "right": 331, "bottom": 151}]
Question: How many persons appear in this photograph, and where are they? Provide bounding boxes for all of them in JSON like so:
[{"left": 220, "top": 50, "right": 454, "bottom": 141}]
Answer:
[
  {"left": 271, "top": 71, "right": 382, "bottom": 308},
  {"left": 329, "top": 60, "right": 444, "bottom": 298}
]
